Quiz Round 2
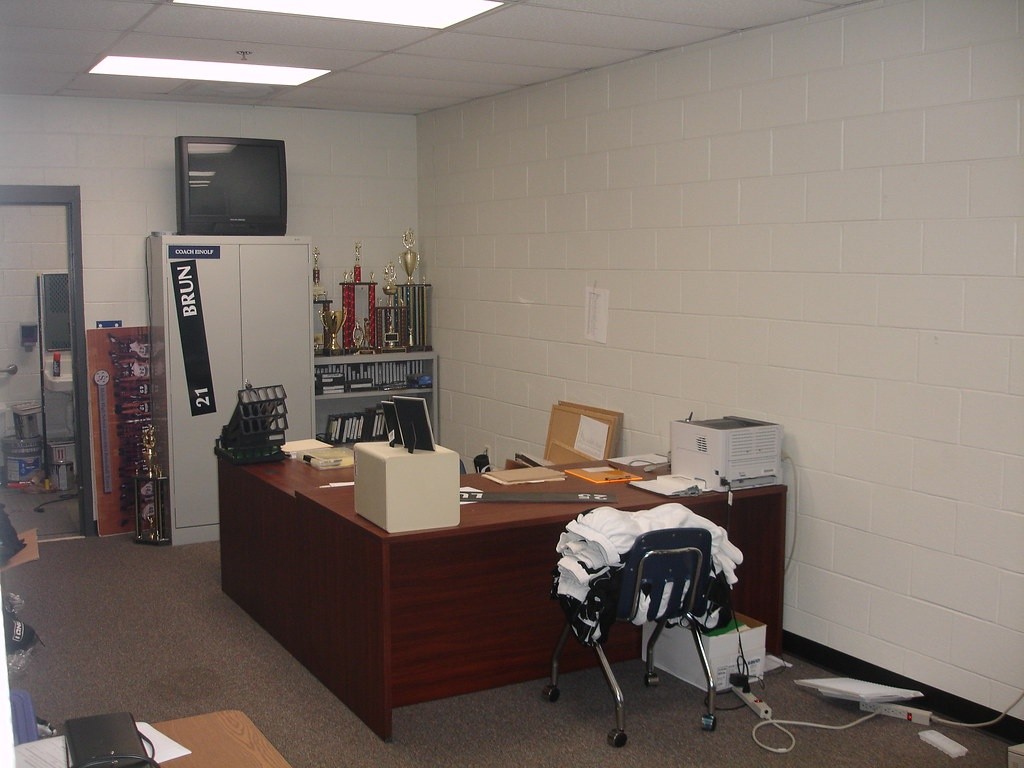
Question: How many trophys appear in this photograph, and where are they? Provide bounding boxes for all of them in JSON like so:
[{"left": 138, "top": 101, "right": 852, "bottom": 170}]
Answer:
[
  {"left": 132, "top": 423, "right": 171, "bottom": 545},
  {"left": 312, "top": 227, "right": 432, "bottom": 357}
]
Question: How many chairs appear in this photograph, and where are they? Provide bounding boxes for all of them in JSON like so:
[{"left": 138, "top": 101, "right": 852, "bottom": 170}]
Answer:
[{"left": 543, "top": 526, "right": 715, "bottom": 748}]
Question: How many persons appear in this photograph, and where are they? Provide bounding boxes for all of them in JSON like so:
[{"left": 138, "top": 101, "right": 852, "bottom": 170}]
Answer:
[{"left": 108, "top": 331, "right": 161, "bottom": 527}]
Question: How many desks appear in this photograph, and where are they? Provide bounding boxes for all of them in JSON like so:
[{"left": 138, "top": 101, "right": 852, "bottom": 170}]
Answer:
[
  {"left": 217, "top": 444, "right": 787, "bottom": 742},
  {"left": 139, "top": 709, "right": 292, "bottom": 768}
]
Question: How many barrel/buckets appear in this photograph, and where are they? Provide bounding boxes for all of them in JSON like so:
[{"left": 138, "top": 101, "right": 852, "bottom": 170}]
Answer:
[
  {"left": 52, "top": 461, "right": 74, "bottom": 491},
  {"left": 13, "top": 413, "right": 39, "bottom": 440},
  {"left": 0, "top": 434, "right": 43, "bottom": 483}
]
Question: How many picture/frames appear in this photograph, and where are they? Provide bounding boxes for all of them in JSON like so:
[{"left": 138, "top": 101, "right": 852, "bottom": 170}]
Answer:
[
  {"left": 381, "top": 401, "right": 405, "bottom": 447},
  {"left": 392, "top": 396, "right": 436, "bottom": 454}
]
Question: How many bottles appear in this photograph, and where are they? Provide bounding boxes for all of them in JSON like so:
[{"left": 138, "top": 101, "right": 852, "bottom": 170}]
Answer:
[
  {"left": 75, "top": 474, "right": 81, "bottom": 492},
  {"left": 53, "top": 351, "right": 60, "bottom": 376}
]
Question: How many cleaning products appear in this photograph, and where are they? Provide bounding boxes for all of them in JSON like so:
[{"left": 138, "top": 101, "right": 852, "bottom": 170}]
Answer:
[{"left": 53, "top": 352, "right": 62, "bottom": 377}]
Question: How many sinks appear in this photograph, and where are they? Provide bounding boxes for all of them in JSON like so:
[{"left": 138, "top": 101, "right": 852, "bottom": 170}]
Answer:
[{"left": 60, "top": 366, "right": 72, "bottom": 378}]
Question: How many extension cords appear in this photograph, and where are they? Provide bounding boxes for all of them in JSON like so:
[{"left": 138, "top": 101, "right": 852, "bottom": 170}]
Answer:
[
  {"left": 731, "top": 685, "right": 771, "bottom": 719},
  {"left": 859, "top": 702, "right": 932, "bottom": 725}
]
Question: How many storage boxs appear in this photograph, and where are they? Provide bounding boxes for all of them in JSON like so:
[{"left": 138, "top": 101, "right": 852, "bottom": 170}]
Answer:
[{"left": 641, "top": 610, "right": 766, "bottom": 692}]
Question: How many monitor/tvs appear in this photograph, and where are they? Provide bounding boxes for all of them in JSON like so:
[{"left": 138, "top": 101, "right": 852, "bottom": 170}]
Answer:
[{"left": 175, "top": 136, "right": 287, "bottom": 236}]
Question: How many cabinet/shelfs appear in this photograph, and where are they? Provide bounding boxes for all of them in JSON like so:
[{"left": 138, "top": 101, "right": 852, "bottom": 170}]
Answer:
[
  {"left": 146, "top": 232, "right": 318, "bottom": 547},
  {"left": 313, "top": 351, "right": 439, "bottom": 444}
]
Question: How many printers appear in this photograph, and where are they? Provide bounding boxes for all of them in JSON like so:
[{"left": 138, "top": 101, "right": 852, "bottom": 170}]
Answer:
[{"left": 670, "top": 416, "right": 783, "bottom": 492}]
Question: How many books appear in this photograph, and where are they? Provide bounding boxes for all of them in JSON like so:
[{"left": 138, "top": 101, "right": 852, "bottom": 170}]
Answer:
[
  {"left": 314, "top": 360, "right": 428, "bottom": 394},
  {"left": 296, "top": 446, "right": 356, "bottom": 471},
  {"left": 325, "top": 404, "right": 387, "bottom": 444}
]
